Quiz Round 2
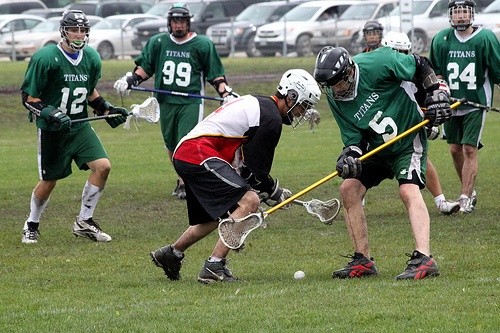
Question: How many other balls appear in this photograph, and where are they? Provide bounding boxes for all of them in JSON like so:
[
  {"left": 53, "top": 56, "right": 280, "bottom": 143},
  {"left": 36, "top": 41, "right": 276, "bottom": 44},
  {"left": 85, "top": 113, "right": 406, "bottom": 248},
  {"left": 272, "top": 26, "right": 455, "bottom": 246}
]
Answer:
[{"left": 294, "top": 271, "right": 305, "bottom": 279}]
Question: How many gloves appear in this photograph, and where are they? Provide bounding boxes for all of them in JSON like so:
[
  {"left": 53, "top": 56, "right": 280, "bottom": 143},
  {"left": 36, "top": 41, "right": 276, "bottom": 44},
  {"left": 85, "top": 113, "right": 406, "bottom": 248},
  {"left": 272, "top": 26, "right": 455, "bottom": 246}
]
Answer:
[
  {"left": 264, "top": 182, "right": 295, "bottom": 209},
  {"left": 218, "top": 88, "right": 238, "bottom": 105},
  {"left": 93, "top": 101, "right": 127, "bottom": 128},
  {"left": 334, "top": 148, "right": 361, "bottom": 172},
  {"left": 40, "top": 105, "right": 71, "bottom": 128},
  {"left": 425, "top": 91, "right": 452, "bottom": 126},
  {"left": 113, "top": 72, "right": 142, "bottom": 95}
]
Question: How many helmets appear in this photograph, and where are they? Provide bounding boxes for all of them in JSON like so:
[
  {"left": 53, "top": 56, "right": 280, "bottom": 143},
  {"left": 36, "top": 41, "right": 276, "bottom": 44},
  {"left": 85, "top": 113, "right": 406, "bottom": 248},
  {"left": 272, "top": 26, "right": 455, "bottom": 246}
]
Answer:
[
  {"left": 60, "top": 9, "right": 90, "bottom": 49},
  {"left": 314, "top": 45, "right": 356, "bottom": 84},
  {"left": 167, "top": 5, "right": 191, "bottom": 37},
  {"left": 362, "top": 20, "right": 384, "bottom": 44},
  {"left": 447, "top": 0, "right": 476, "bottom": 30}
]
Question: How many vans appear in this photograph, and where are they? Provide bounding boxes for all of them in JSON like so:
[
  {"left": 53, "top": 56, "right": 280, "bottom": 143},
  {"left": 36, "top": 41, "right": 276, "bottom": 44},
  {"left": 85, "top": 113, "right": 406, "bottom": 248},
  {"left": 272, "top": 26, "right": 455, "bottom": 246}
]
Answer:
[
  {"left": 374, "top": 0, "right": 494, "bottom": 56},
  {"left": 254, "top": 0, "right": 360, "bottom": 57},
  {"left": 309, "top": 0, "right": 400, "bottom": 57},
  {"left": 129, "top": 0, "right": 269, "bottom": 61},
  {"left": 205, "top": 0, "right": 309, "bottom": 58}
]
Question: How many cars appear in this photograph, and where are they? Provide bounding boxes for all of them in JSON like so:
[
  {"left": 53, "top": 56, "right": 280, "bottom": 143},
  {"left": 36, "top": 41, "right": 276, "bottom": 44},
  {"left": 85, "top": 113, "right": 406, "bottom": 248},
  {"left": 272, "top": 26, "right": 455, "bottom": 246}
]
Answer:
[
  {"left": 0, "top": 0, "right": 188, "bottom": 61},
  {"left": 470, "top": 0, "right": 500, "bottom": 45}
]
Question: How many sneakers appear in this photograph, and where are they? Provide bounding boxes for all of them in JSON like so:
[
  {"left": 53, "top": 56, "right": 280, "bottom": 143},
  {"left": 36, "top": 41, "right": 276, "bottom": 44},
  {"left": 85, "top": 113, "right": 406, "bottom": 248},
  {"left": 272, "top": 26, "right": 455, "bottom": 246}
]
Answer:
[
  {"left": 21, "top": 221, "right": 40, "bottom": 243},
  {"left": 150, "top": 242, "right": 185, "bottom": 282},
  {"left": 330, "top": 253, "right": 380, "bottom": 279},
  {"left": 436, "top": 199, "right": 460, "bottom": 216},
  {"left": 456, "top": 188, "right": 478, "bottom": 213},
  {"left": 397, "top": 251, "right": 441, "bottom": 282},
  {"left": 197, "top": 257, "right": 240, "bottom": 285},
  {"left": 72, "top": 217, "right": 112, "bottom": 241}
]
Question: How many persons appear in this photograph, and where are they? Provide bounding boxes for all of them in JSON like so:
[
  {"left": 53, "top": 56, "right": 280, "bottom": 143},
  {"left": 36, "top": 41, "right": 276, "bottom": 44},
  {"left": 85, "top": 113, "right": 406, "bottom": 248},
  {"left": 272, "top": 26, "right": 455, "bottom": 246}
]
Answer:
[
  {"left": 360, "top": 22, "right": 459, "bottom": 215},
  {"left": 113, "top": 3, "right": 240, "bottom": 199},
  {"left": 313, "top": 45, "right": 450, "bottom": 281},
  {"left": 429, "top": 0, "right": 500, "bottom": 213},
  {"left": 149, "top": 68, "right": 322, "bottom": 283},
  {"left": 19, "top": 10, "right": 129, "bottom": 244}
]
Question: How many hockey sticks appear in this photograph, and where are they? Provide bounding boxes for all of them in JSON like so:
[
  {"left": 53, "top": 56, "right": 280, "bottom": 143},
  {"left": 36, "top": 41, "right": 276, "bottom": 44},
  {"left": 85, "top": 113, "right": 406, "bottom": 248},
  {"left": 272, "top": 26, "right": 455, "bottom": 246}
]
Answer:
[
  {"left": 54, "top": 97, "right": 161, "bottom": 129},
  {"left": 450, "top": 97, "right": 500, "bottom": 112},
  {"left": 293, "top": 198, "right": 341, "bottom": 224},
  {"left": 218, "top": 98, "right": 467, "bottom": 249},
  {"left": 130, "top": 84, "right": 321, "bottom": 125}
]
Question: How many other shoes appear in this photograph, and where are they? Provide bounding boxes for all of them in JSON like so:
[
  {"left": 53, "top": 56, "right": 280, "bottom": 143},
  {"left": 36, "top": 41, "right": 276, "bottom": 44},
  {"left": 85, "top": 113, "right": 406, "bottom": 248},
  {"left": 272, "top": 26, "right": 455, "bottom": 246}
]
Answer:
[{"left": 173, "top": 177, "right": 188, "bottom": 197}]
